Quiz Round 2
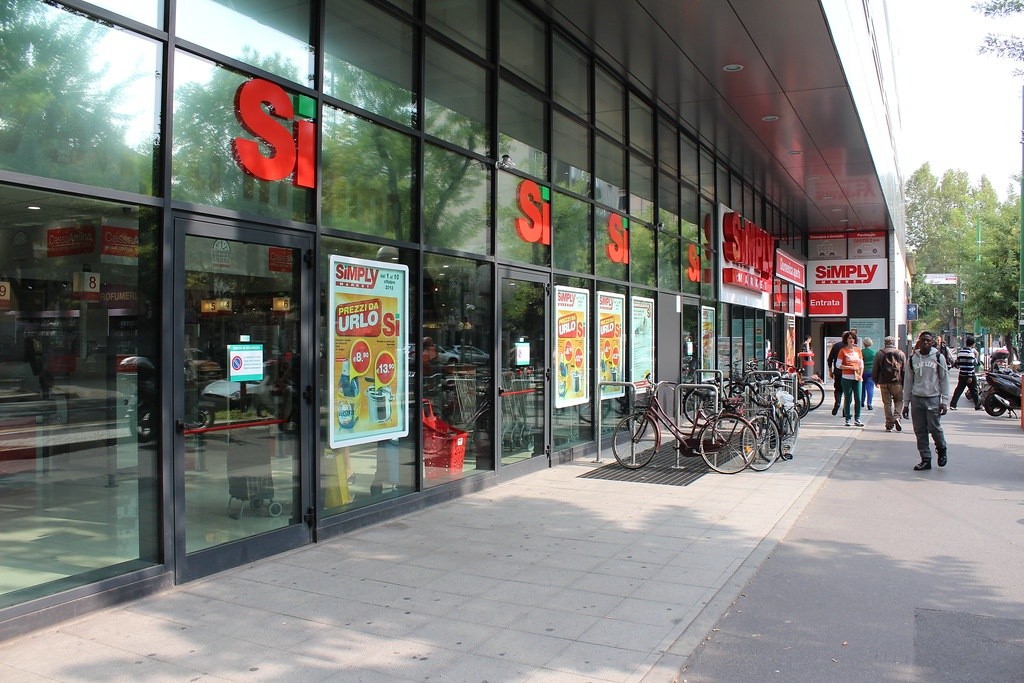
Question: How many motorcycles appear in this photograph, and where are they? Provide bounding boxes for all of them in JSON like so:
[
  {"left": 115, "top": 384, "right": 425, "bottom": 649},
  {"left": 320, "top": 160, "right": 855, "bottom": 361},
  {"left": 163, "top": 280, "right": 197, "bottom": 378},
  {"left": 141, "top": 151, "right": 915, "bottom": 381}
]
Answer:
[
  {"left": 116, "top": 355, "right": 215, "bottom": 444},
  {"left": 974, "top": 349, "right": 1022, "bottom": 420}
]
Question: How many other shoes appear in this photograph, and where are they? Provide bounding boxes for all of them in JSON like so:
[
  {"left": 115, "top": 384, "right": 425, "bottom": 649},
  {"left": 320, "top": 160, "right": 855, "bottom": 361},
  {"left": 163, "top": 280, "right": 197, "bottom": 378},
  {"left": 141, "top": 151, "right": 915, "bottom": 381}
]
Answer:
[
  {"left": 894, "top": 418, "right": 902, "bottom": 431},
  {"left": 868, "top": 406, "right": 873, "bottom": 409},
  {"left": 913, "top": 460, "right": 931, "bottom": 471},
  {"left": 845, "top": 420, "right": 850, "bottom": 425},
  {"left": 886, "top": 426, "right": 893, "bottom": 432},
  {"left": 950, "top": 406, "right": 957, "bottom": 410},
  {"left": 861, "top": 404, "right": 864, "bottom": 407},
  {"left": 842, "top": 409, "right": 846, "bottom": 417},
  {"left": 854, "top": 420, "right": 864, "bottom": 426},
  {"left": 937, "top": 447, "right": 947, "bottom": 467},
  {"left": 832, "top": 407, "right": 839, "bottom": 415},
  {"left": 975, "top": 406, "right": 983, "bottom": 410}
]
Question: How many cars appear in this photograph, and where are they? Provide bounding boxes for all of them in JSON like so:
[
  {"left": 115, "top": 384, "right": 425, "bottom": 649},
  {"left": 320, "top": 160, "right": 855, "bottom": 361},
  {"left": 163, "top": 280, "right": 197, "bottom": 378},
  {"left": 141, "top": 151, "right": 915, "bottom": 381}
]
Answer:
[
  {"left": 199, "top": 359, "right": 281, "bottom": 411},
  {"left": 407, "top": 343, "right": 461, "bottom": 371},
  {"left": 451, "top": 344, "right": 491, "bottom": 368}
]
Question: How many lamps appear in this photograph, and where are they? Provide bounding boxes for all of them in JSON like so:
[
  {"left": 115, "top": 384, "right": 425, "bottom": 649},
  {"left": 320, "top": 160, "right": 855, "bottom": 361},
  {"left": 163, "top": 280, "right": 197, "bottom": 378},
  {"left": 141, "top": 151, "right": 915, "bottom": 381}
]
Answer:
[{"left": 495, "top": 155, "right": 515, "bottom": 170}]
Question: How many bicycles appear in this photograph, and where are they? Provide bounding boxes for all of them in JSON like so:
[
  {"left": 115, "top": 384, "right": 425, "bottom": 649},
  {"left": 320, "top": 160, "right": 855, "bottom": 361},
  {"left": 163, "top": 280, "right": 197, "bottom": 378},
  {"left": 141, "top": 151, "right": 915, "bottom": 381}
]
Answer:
[
  {"left": 407, "top": 370, "right": 612, "bottom": 453},
  {"left": 682, "top": 357, "right": 825, "bottom": 472},
  {"left": 610, "top": 372, "right": 758, "bottom": 475}
]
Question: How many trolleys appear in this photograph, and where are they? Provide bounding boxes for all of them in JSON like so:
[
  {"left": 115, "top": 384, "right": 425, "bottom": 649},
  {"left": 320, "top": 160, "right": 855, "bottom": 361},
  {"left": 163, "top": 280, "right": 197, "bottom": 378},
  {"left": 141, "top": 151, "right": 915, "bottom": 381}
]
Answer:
[{"left": 223, "top": 419, "right": 281, "bottom": 520}]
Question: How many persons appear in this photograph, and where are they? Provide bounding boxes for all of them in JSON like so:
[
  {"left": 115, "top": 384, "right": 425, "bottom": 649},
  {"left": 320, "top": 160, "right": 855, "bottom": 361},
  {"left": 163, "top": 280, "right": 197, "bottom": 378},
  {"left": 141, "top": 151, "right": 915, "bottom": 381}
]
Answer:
[
  {"left": 24, "top": 336, "right": 46, "bottom": 392},
  {"left": 860, "top": 337, "right": 877, "bottom": 410},
  {"left": 800, "top": 335, "right": 812, "bottom": 361},
  {"left": 827, "top": 331, "right": 849, "bottom": 418},
  {"left": 901, "top": 331, "right": 950, "bottom": 470},
  {"left": 872, "top": 336, "right": 907, "bottom": 432},
  {"left": 836, "top": 332, "right": 864, "bottom": 427},
  {"left": 910, "top": 336, "right": 1019, "bottom": 371},
  {"left": 950, "top": 337, "right": 985, "bottom": 411},
  {"left": 258, "top": 329, "right": 293, "bottom": 432},
  {"left": 423, "top": 337, "right": 435, "bottom": 384}
]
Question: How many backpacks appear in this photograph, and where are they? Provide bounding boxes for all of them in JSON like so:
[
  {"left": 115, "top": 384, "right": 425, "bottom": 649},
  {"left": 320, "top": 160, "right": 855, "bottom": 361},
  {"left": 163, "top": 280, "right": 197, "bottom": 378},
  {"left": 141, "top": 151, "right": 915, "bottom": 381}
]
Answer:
[{"left": 879, "top": 349, "right": 900, "bottom": 383}]
